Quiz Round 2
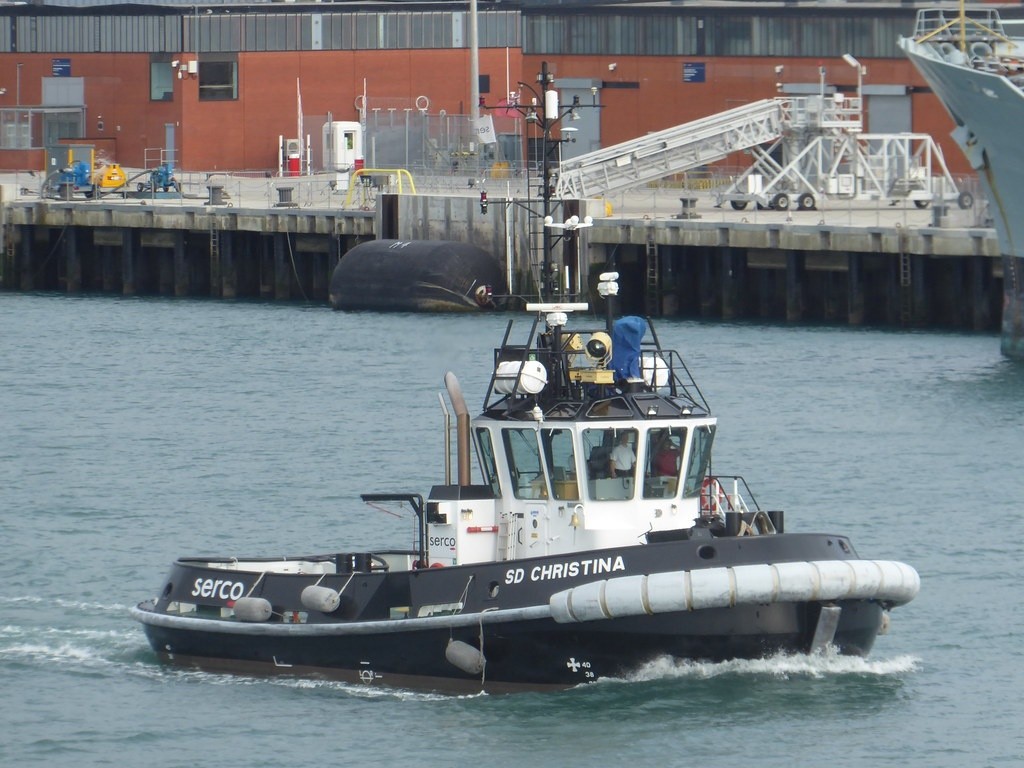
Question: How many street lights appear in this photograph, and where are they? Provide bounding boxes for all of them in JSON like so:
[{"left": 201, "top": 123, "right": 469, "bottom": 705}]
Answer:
[
  {"left": 16, "top": 63, "right": 23, "bottom": 106},
  {"left": 841, "top": 53, "right": 864, "bottom": 134},
  {"left": 484, "top": 58, "right": 607, "bottom": 302}
]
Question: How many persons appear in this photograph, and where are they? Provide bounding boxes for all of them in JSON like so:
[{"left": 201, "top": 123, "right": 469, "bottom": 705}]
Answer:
[
  {"left": 610, "top": 432, "right": 636, "bottom": 479},
  {"left": 654, "top": 432, "right": 683, "bottom": 476}
]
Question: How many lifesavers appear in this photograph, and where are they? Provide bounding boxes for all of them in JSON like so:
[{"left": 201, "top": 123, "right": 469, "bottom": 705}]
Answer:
[{"left": 701, "top": 478, "right": 723, "bottom": 512}]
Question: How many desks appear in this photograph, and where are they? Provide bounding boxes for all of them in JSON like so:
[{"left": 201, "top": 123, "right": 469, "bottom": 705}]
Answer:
[{"left": 540, "top": 481, "right": 579, "bottom": 501}]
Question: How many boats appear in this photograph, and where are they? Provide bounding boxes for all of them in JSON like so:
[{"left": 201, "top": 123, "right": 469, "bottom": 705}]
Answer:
[
  {"left": 131, "top": 217, "right": 923, "bottom": 688},
  {"left": 897, "top": 5, "right": 1024, "bottom": 367}
]
